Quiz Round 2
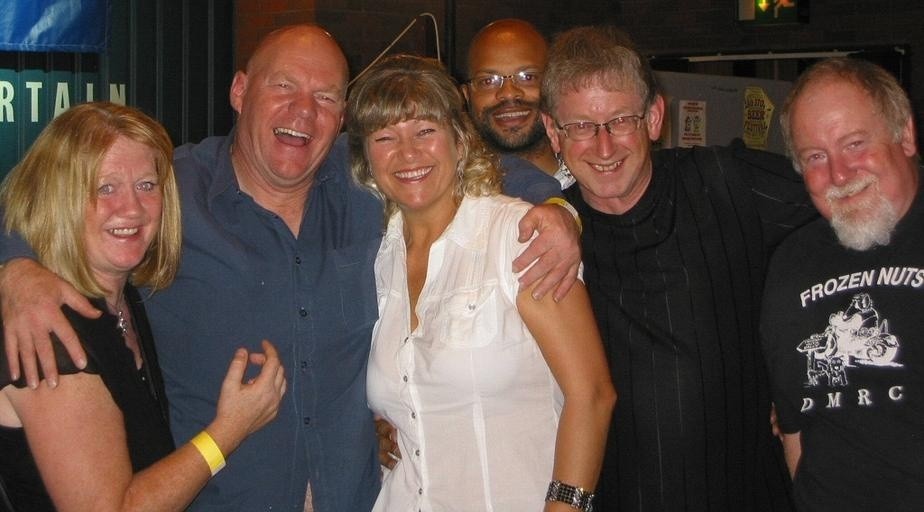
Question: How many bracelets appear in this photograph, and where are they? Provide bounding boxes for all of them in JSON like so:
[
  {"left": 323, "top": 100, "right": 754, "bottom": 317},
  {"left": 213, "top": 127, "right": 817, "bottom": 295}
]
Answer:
[
  {"left": 185, "top": 428, "right": 225, "bottom": 477},
  {"left": 541, "top": 195, "right": 583, "bottom": 237},
  {"left": 541, "top": 479, "right": 594, "bottom": 512}
]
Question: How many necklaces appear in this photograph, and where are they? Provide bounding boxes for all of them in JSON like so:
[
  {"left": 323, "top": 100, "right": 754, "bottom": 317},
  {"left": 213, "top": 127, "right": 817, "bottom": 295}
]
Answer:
[{"left": 102, "top": 290, "right": 127, "bottom": 336}]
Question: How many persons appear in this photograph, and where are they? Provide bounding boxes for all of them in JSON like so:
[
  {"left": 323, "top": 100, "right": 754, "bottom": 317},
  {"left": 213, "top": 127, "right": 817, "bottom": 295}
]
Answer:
[
  {"left": 537, "top": 23, "right": 833, "bottom": 512},
  {"left": 1, "top": 22, "right": 585, "bottom": 512},
  {"left": 751, "top": 56, "right": 923, "bottom": 510},
  {"left": 1, "top": 100, "right": 289, "bottom": 511},
  {"left": 461, "top": 20, "right": 576, "bottom": 192},
  {"left": 343, "top": 51, "right": 618, "bottom": 511}
]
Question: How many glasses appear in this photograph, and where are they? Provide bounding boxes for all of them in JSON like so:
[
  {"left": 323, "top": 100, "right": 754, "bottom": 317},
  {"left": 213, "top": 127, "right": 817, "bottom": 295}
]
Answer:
[
  {"left": 554, "top": 102, "right": 648, "bottom": 142},
  {"left": 462, "top": 70, "right": 544, "bottom": 94}
]
